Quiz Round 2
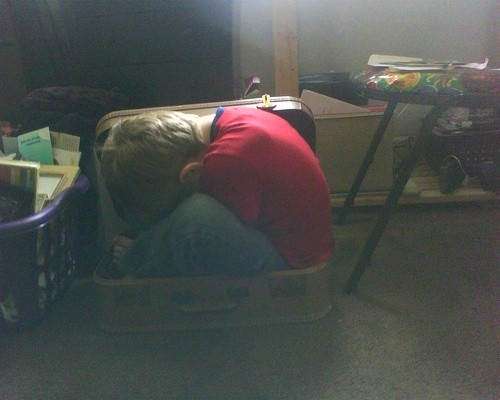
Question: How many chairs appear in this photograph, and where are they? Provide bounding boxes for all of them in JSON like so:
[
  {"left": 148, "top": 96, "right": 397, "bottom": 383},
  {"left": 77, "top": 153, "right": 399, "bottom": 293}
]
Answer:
[{"left": 336, "top": 58, "right": 498, "bottom": 290}]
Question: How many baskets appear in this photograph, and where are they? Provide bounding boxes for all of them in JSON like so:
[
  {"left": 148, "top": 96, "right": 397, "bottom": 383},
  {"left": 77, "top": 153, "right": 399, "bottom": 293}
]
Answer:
[{"left": 1, "top": 175, "right": 91, "bottom": 331}]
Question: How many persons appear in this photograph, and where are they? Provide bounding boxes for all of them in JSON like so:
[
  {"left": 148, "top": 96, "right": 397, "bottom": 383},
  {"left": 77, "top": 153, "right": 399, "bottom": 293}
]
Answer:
[{"left": 98, "top": 105, "right": 338, "bottom": 280}]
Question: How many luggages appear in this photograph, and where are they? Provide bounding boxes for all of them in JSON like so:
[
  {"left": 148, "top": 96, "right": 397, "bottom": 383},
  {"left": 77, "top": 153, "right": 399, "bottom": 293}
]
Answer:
[{"left": 92, "top": 94, "right": 333, "bottom": 334}]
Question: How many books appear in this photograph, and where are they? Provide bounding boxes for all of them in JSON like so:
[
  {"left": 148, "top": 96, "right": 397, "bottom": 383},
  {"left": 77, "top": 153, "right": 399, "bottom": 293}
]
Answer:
[{"left": 0, "top": 160, "right": 40, "bottom": 223}]
